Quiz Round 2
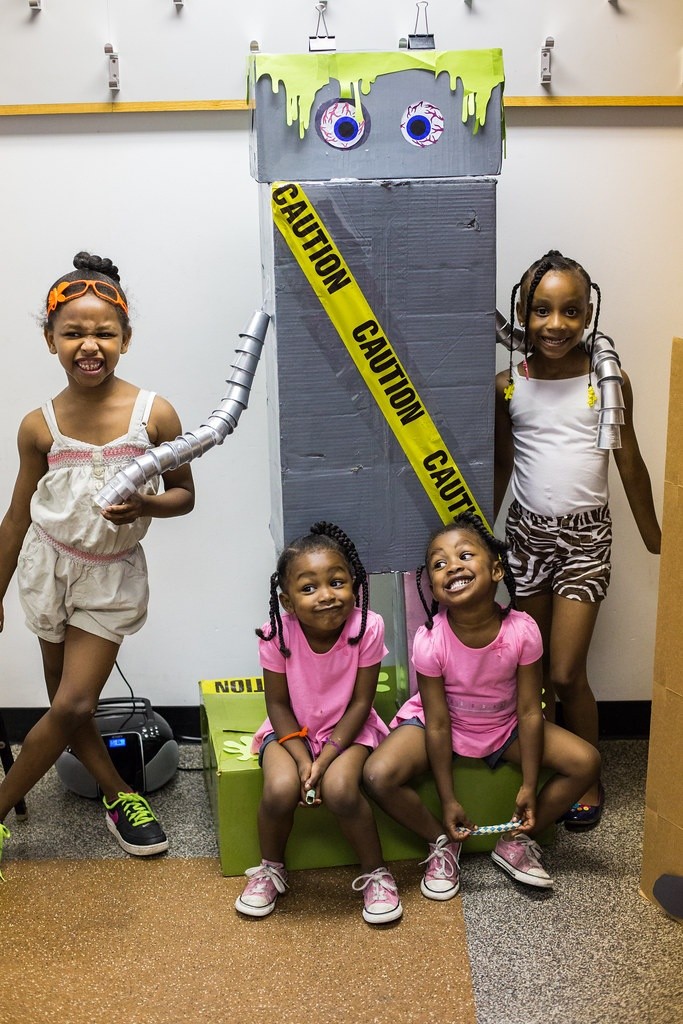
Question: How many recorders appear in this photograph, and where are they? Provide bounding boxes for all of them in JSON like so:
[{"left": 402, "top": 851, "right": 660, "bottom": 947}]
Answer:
[{"left": 55, "top": 697, "right": 178, "bottom": 799}]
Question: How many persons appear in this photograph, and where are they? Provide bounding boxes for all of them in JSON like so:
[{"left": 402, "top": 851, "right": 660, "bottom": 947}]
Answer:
[
  {"left": 0, "top": 252, "right": 195, "bottom": 855},
  {"left": 363, "top": 510, "right": 603, "bottom": 901},
  {"left": 233, "top": 520, "right": 403, "bottom": 924},
  {"left": 495, "top": 249, "right": 663, "bottom": 831}
]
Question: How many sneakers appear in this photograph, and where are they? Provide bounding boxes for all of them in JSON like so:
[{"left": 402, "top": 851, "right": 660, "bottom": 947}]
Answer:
[
  {"left": 235, "top": 858, "right": 290, "bottom": 916},
  {"left": 0, "top": 822, "right": 11, "bottom": 881},
  {"left": 352, "top": 867, "right": 403, "bottom": 923},
  {"left": 491, "top": 832, "right": 553, "bottom": 887},
  {"left": 418, "top": 834, "right": 462, "bottom": 900},
  {"left": 102, "top": 790, "right": 169, "bottom": 855}
]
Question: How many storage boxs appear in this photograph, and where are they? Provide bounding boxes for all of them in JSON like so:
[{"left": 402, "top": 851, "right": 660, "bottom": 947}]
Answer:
[{"left": 200, "top": 49, "right": 542, "bottom": 877}]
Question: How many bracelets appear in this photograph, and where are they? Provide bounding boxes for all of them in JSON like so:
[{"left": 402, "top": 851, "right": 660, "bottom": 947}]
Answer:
[
  {"left": 278, "top": 728, "right": 308, "bottom": 743},
  {"left": 322, "top": 737, "right": 342, "bottom": 754}
]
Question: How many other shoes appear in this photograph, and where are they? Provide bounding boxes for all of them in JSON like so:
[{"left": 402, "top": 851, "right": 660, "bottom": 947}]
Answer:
[{"left": 566, "top": 778, "right": 603, "bottom": 824}]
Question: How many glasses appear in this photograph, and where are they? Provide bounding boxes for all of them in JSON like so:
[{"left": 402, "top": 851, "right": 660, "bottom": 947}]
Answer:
[{"left": 48, "top": 279, "right": 128, "bottom": 325}]
